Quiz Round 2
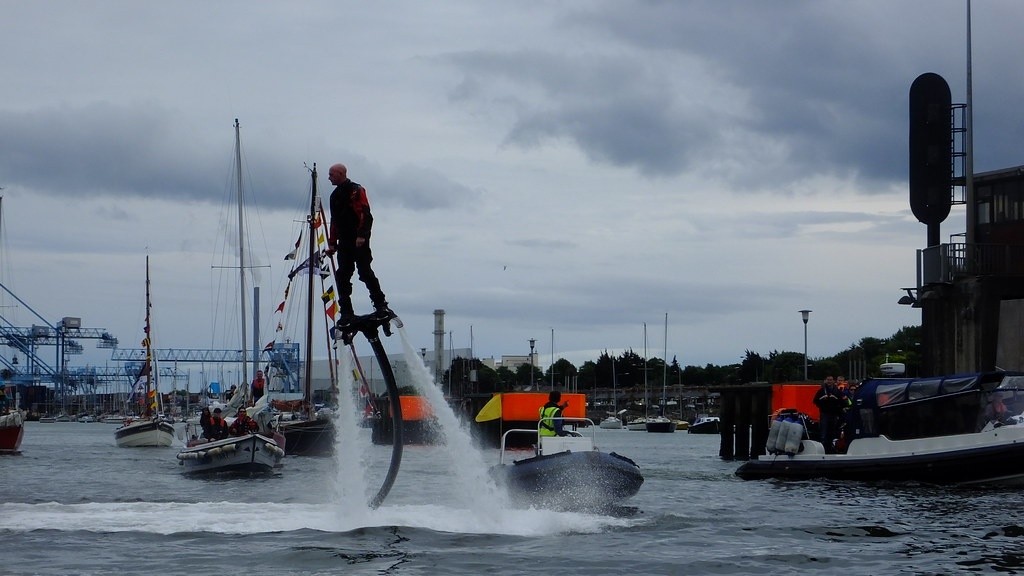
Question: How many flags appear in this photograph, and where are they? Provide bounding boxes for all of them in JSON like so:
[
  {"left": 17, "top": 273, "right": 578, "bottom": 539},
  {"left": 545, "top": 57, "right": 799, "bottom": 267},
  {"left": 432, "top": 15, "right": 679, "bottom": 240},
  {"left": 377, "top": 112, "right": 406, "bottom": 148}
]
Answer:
[
  {"left": 141, "top": 279, "right": 160, "bottom": 411},
  {"left": 263, "top": 197, "right": 376, "bottom": 420}
]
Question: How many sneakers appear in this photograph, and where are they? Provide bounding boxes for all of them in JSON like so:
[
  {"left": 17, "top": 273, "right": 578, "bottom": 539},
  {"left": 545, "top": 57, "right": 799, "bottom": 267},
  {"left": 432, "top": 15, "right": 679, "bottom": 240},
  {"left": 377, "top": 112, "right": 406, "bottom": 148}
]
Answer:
[
  {"left": 338, "top": 314, "right": 356, "bottom": 327},
  {"left": 377, "top": 307, "right": 389, "bottom": 321}
]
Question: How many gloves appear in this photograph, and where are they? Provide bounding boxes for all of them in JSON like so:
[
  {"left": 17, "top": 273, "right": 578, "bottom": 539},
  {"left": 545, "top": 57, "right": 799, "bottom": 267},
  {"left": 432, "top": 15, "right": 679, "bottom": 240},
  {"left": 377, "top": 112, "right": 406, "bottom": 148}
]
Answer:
[{"left": 210, "top": 438, "right": 216, "bottom": 442}]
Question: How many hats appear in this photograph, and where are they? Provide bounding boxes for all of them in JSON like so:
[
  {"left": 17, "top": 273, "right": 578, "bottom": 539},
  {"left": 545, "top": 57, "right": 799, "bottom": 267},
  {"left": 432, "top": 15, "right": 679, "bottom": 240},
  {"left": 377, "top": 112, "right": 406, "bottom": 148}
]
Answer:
[{"left": 214, "top": 408, "right": 222, "bottom": 413}]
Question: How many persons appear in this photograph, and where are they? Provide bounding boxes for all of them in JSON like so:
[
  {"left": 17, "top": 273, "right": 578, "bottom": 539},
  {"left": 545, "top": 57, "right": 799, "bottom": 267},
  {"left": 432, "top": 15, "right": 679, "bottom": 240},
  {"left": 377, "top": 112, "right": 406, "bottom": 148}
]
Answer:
[
  {"left": 813, "top": 375, "right": 856, "bottom": 454},
  {"left": 202, "top": 370, "right": 264, "bottom": 442},
  {"left": 538, "top": 391, "right": 581, "bottom": 437},
  {"left": 328, "top": 164, "right": 389, "bottom": 328}
]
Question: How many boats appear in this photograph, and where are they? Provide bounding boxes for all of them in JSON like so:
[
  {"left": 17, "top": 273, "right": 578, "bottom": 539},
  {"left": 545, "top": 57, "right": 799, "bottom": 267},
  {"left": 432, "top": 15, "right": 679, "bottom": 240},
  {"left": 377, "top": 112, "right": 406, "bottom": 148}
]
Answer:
[
  {"left": 671, "top": 419, "right": 689, "bottom": 430},
  {"left": 600, "top": 417, "right": 622, "bottom": 429},
  {"left": 627, "top": 418, "right": 647, "bottom": 431},
  {"left": 646, "top": 416, "right": 678, "bottom": 433},
  {"left": 734, "top": 371, "right": 1024, "bottom": 487},
  {"left": 688, "top": 417, "right": 721, "bottom": 434},
  {"left": 486, "top": 418, "right": 644, "bottom": 512}
]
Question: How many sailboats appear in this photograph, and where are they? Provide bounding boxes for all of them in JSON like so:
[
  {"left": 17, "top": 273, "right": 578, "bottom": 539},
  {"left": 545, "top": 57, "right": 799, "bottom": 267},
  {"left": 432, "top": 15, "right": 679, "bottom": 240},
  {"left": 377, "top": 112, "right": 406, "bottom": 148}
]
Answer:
[
  {"left": 0, "top": 185, "right": 25, "bottom": 451},
  {"left": 38, "top": 356, "right": 380, "bottom": 429},
  {"left": 256, "top": 161, "right": 342, "bottom": 457},
  {"left": 175, "top": 118, "right": 287, "bottom": 477},
  {"left": 114, "top": 255, "right": 175, "bottom": 447}
]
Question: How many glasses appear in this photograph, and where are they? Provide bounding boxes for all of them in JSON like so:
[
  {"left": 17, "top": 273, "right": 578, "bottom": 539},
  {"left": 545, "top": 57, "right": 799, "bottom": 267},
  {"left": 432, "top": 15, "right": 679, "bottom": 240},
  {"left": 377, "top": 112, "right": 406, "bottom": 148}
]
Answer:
[{"left": 239, "top": 411, "right": 246, "bottom": 413}]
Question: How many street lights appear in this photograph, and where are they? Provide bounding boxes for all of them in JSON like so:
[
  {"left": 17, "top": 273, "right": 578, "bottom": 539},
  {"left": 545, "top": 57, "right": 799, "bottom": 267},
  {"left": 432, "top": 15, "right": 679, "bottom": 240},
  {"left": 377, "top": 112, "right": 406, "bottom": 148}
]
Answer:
[
  {"left": 799, "top": 310, "right": 813, "bottom": 381},
  {"left": 527, "top": 337, "right": 538, "bottom": 391}
]
